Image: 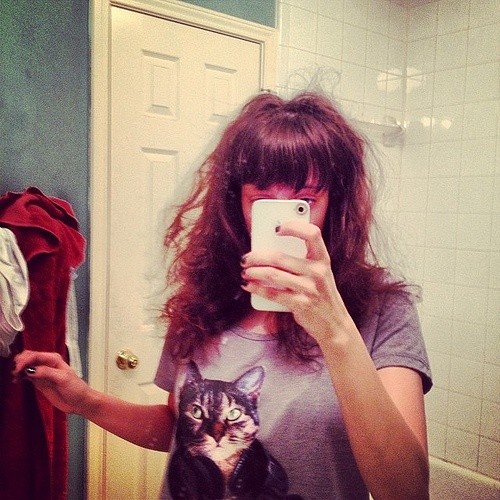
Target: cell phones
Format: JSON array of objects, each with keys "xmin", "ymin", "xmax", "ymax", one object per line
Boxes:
[{"xmin": 251, "ymin": 199, "xmax": 311, "ymax": 312}]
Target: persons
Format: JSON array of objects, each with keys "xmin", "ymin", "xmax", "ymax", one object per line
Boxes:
[{"xmin": 11, "ymin": 92, "xmax": 433, "ymax": 500}]
